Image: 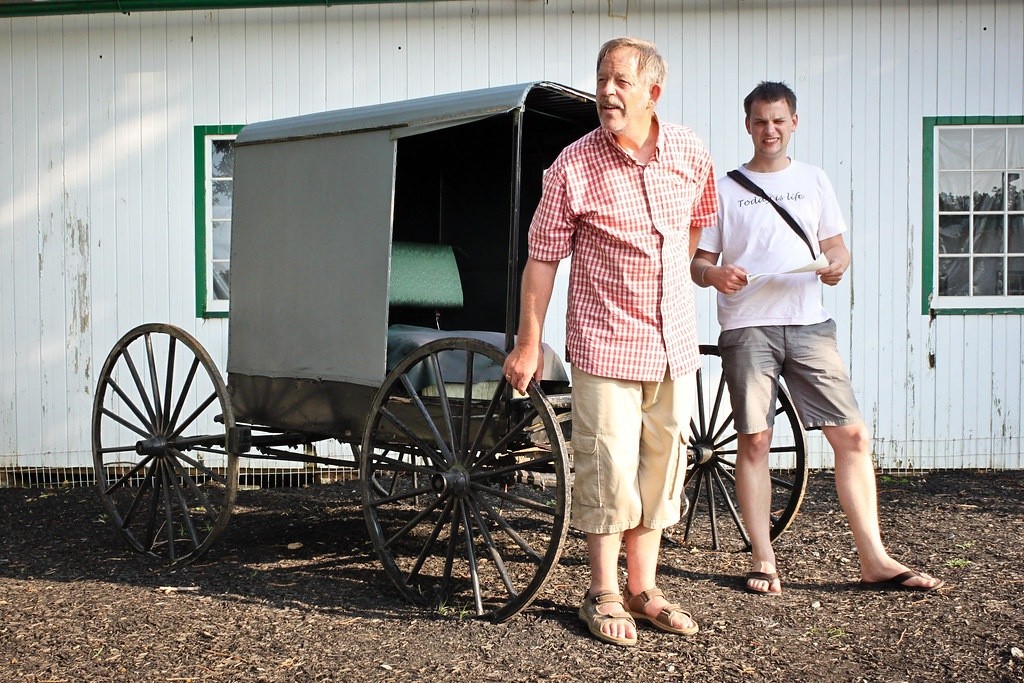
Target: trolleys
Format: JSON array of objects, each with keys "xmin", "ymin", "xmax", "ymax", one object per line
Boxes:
[{"xmin": 89, "ymin": 79, "xmax": 808, "ymax": 629}]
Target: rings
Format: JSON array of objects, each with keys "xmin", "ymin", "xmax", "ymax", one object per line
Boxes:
[{"xmin": 506, "ymin": 373, "xmax": 511, "ymax": 378}]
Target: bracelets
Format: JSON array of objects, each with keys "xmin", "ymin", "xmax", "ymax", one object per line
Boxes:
[{"xmin": 702, "ymin": 266, "xmax": 715, "ymax": 287}]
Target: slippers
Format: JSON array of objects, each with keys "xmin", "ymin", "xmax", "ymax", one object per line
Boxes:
[
  {"xmin": 859, "ymin": 566, "xmax": 944, "ymax": 591},
  {"xmin": 745, "ymin": 571, "xmax": 782, "ymax": 596}
]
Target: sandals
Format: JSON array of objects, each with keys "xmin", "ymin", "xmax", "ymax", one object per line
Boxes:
[
  {"xmin": 622, "ymin": 580, "xmax": 700, "ymax": 635},
  {"xmin": 578, "ymin": 588, "xmax": 638, "ymax": 646}
]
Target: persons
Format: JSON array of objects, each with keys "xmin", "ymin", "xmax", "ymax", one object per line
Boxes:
[
  {"xmin": 502, "ymin": 39, "xmax": 717, "ymax": 645},
  {"xmin": 691, "ymin": 81, "xmax": 944, "ymax": 596}
]
